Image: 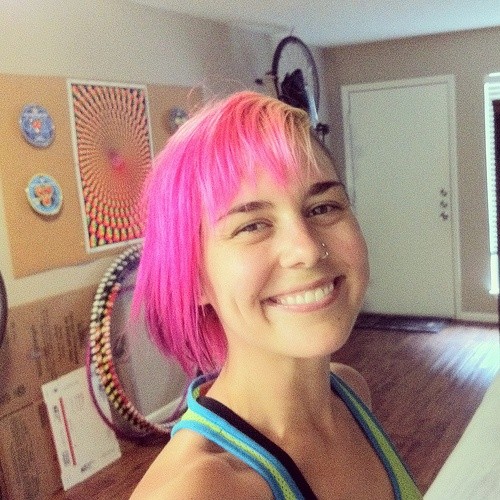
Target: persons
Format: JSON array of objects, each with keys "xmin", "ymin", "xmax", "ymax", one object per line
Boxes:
[{"xmin": 126, "ymin": 92, "xmax": 425, "ymax": 500}]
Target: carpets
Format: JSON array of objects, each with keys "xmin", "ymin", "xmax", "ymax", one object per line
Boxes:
[{"xmin": 355, "ymin": 311, "xmax": 451, "ymax": 334}]
